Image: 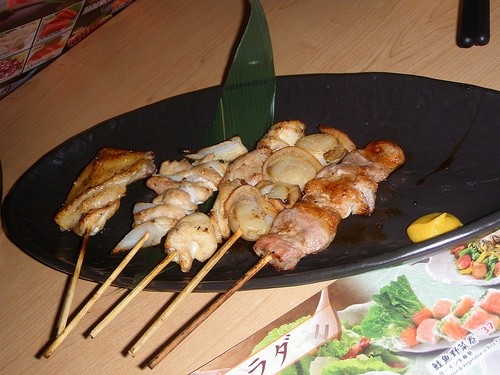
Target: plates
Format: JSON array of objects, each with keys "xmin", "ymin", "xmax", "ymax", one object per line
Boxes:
[
  {"xmin": 337, "ymin": 285, "xmax": 500, "ymax": 353},
  {"xmin": 0, "ymin": 73, "xmax": 500, "ymax": 293},
  {"xmin": 426, "ymin": 233, "xmax": 500, "ymax": 286}
]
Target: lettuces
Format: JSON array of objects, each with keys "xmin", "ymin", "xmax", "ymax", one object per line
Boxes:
[{"xmin": 249, "ymin": 276, "xmax": 429, "ymax": 375}]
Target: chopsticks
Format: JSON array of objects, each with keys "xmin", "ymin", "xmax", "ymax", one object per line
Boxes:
[{"xmin": 458, "ymin": 0, "xmax": 490, "ymax": 48}]
